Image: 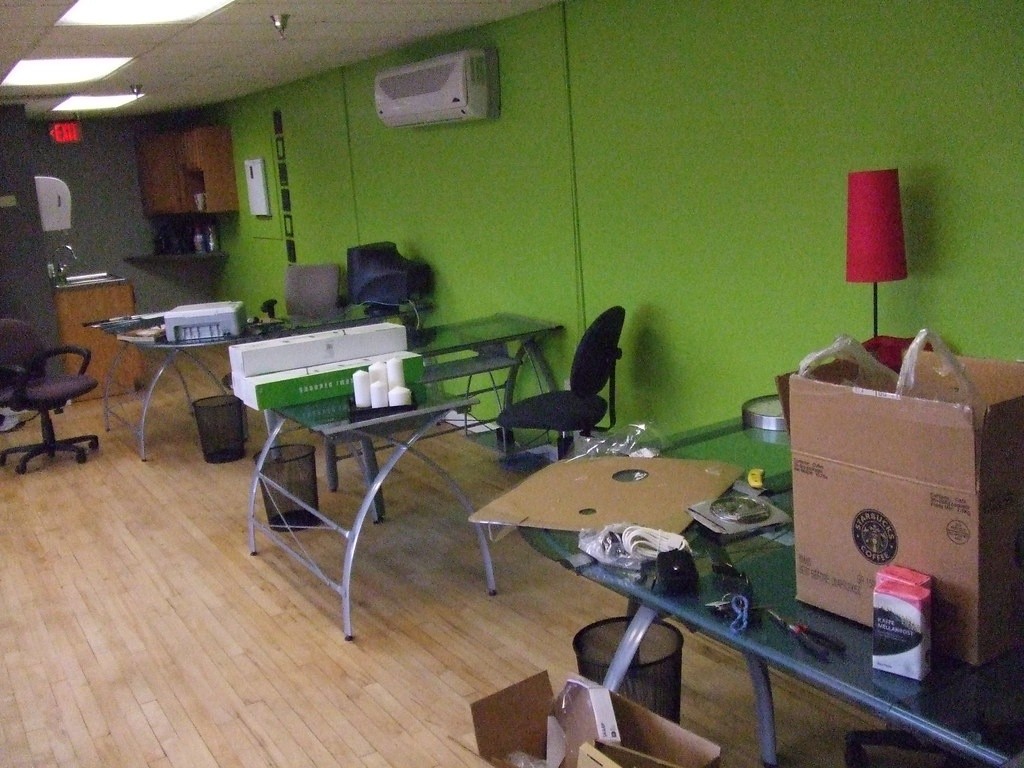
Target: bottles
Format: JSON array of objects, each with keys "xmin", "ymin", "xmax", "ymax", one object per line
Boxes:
[
  {"xmin": 194, "ymin": 226, "xmax": 206, "ymax": 254},
  {"xmin": 207, "ymin": 217, "xmax": 220, "ymax": 252}
]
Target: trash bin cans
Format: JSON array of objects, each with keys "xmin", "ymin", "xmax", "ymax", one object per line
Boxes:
[
  {"xmin": 251, "ymin": 444, "xmax": 321, "ymax": 531},
  {"xmin": 193, "ymin": 395, "xmax": 244, "ymax": 463},
  {"xmin": 574, "ymin": 616, "xmax": 683, "ymax": 722}
]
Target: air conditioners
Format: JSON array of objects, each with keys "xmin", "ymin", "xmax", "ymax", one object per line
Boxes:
[{"xmin": 374, "ymin": 46, "xmax": 500, "ymax": 128}]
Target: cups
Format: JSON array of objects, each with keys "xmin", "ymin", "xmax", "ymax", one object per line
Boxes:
[{"xmin": 194, "ymin": 194, "xmax": 205, "ymax": 210}]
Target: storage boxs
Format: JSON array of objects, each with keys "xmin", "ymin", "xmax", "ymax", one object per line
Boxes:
[
  {"xmin": 230, "ymin": 322, "xmax": 425, "ymax": 410},
  {"xmin": 773, "ymin": 350, "xmax": 1024, "ymax": 665},
  {"xmin": 447, "ymin": 669, "xmax": 722, "ymax": 768}
]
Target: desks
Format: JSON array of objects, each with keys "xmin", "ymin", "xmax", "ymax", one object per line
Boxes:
[
  {"xmin": 103, "ymin": 334, "xmax": 239, "ymax": 462},
  {"xmin": 304, "ymin": 313, "xmax": 569, "ymax": 523},
  {"xmin": 247, "ymin": 383, "xmax": 498, "ymax": 642},
  {"xmin": 516, "ymin": 415, "xmax": 1023, "ymax": 768}
]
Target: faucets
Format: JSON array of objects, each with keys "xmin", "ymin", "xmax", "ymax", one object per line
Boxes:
[{"xmin": 54, "ymin": 245, "xmax": 78, "ymax": 281}]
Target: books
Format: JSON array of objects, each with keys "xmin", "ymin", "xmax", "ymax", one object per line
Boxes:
[{"xmin": 116, "ymin": 324, "xmax": 166, "ymax": 342}]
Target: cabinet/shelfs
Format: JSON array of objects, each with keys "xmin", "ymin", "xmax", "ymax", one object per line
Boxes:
[
  {"xmin": 139, "ymin": 123, "xmax": 238, "ymax": 215},
  {"xmin": 54, "ymin": 272, "xmax": 146, "ymax": 403}
]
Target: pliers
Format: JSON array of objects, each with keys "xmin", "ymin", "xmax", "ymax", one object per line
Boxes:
[{"xmin": 767, "ymin": 607, "xmax": 847, "ymax": 658}]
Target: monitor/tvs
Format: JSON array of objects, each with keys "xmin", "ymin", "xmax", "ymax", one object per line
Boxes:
[{"xmin": 347, "ymin": 241, "xmax": 433, "ymax": 317}]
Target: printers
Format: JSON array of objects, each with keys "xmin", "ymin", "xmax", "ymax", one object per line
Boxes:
[{"xmin": 163, "ymin": 301, "xmax": 247, "ymax": 344}]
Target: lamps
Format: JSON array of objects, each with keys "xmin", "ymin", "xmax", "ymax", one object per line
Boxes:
[{"xmin": 847, "ymin": 168, "xmax": 909, "ymax": 337}]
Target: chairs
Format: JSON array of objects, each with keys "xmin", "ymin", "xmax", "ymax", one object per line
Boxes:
[
  {"xmin": 496, "ymin": 305, "xmax": 626, "ymax": 459},
  {"xmin": 0, "ymin": 318, "xmax": 98, "ymax": 474},
  {"xmin": 261, "ymin": 264, "xmax": 342, "ymax": 330}
]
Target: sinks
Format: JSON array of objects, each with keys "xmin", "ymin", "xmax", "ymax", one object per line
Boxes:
[{"xmin": 65, "ymin": 272, "xmax": 125, "ymax": 286}]
